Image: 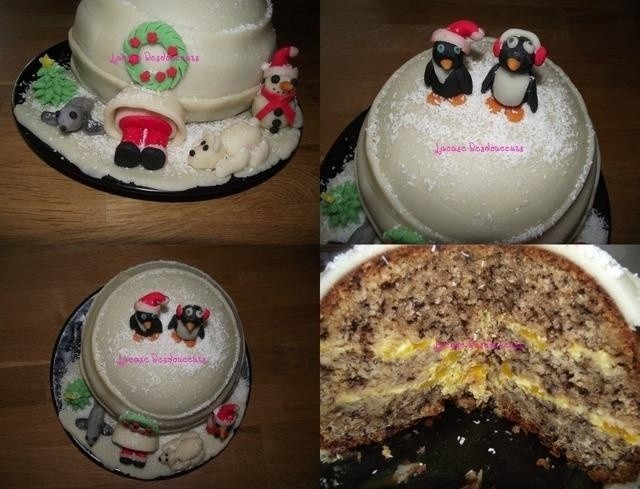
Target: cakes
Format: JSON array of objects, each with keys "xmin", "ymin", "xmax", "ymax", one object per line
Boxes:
[
  {"xmin": 12, "ymin": 0, "xmax": 304, "ymax": 193},
  {"xmin": 320, "ymin": 245, "xmax": 640, "ymax": 489},
  {"xmin": 321, "ymin": 18, "xmax": 603, "ymax": 245},
  {"xmin": 57, "ymin": 260, "xmax": 251, "ymax": 480}
]
